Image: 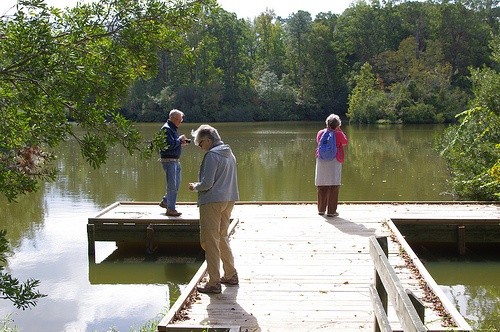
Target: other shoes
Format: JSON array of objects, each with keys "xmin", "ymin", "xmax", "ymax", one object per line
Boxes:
[
  {"xmin": 319, "ymin": 212, "xmax": 339, "ymax": 216},
  {"xmin": 158, "ymin": 201, "xmax": 167, "ymax": 209},
  {"xmin": 166, "ymin": 209, "xmax": 182, "ymax": 215}
]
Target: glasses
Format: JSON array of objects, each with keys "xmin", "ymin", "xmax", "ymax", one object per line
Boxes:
[{"xmin": 171, "ymin": 117, "xmax": 184, "ymax": 122}]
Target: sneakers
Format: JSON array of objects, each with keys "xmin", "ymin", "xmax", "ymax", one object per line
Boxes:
[
  {"xmin": 220, "ymin": 277, "xmax": 238, "ymax": 285},
  {"xmin": 196, "ymin": 283, "xmax": 221, "ymax": 293}
]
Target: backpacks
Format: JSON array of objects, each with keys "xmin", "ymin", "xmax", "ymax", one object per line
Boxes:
[{"xmin": 319, "ymin": 128, "xmax": 337, "ymax": 161}]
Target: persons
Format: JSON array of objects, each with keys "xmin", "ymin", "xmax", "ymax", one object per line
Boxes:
[
  {"xmin": 314, "ymin": 113, "xmax": 350, "ymax": 217},
  {"xmin": 158, "ymin": 109, "xmax": 189, "ymax": 217},
  {"xmin": 188, "ymin": 123, "xmax": 239, "ymax": 294}
]
